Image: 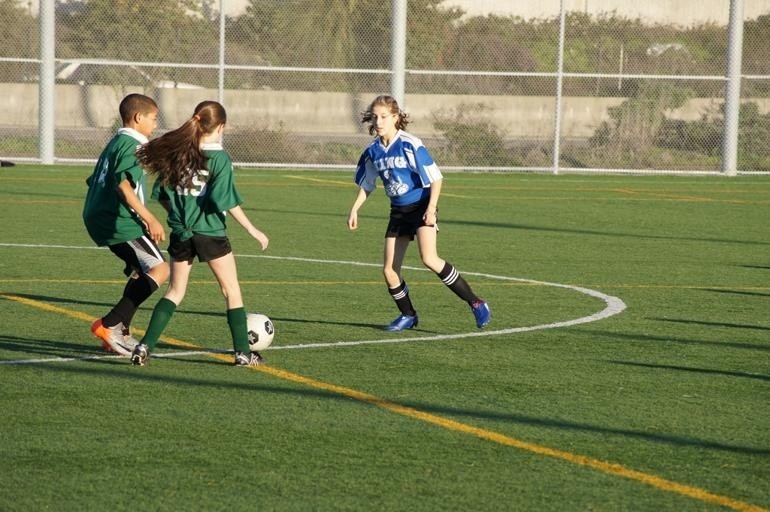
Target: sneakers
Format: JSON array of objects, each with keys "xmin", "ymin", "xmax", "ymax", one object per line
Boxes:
[
  {"xmin": 381, "ymin": 313, "xmax": 419, "ymax": 332},
  {"xmin": 130, "ymin": 344, "xmax": 151, "ymax": 368},
  {"xmin": 234, "ymin": 350, "xmax": 270, "ymax": 367},
  {"xmin": 90, "ymin": 317, "xmax": 141, "ymax": 355},
  {"xmin": 472, "ymin": 300, "xmax": 492, "ymax": 329}
]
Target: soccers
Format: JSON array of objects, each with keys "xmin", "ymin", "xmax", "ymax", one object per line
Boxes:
[{"xmin": 246, "ymin": 313, "xmax": 274, "ymax": 351}]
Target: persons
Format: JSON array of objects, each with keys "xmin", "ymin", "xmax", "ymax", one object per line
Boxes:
[
  {"xmin": 81, "ymin": 92, "xmax": 174, "ymax": 360},
  {"xmin": 130, "ymin": 99, "xmax": 272, "ymax": 366},
  {"xmin": 347, "ymin": 94, "xmax": 493, "ymax": 330}
]
